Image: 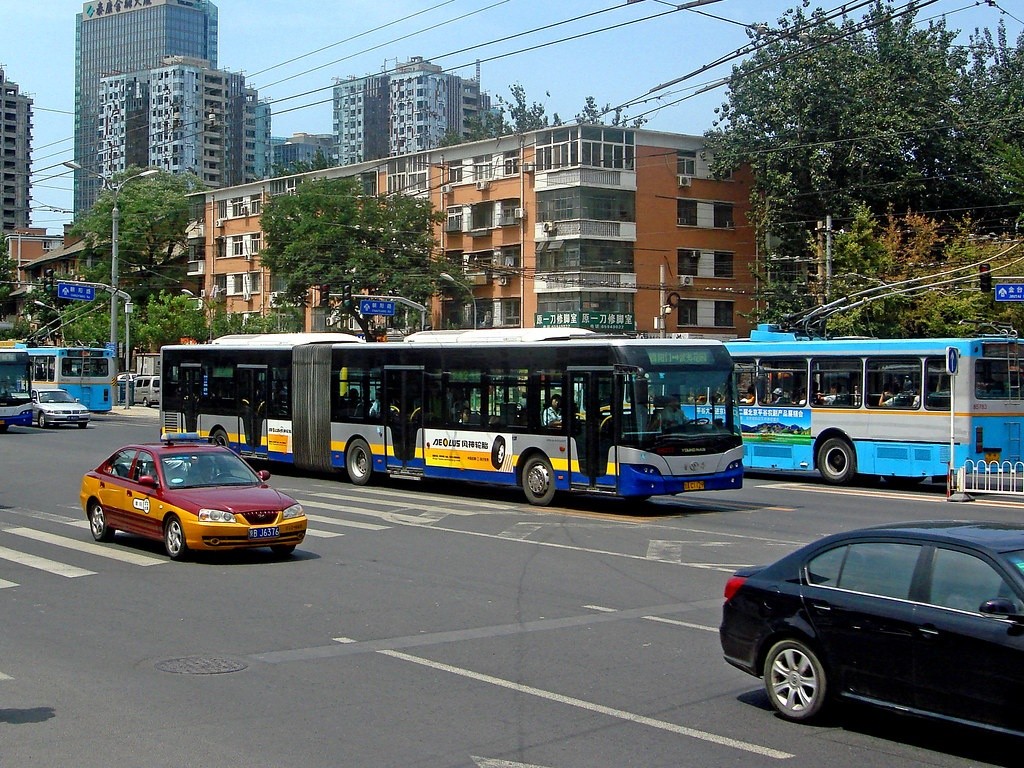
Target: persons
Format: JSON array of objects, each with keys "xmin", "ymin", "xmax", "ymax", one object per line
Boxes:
[
  {"xmin": 347, "ymin": 388, "xmax": 359, "ymax": 406},
  {"xmin": 543, "ymin": 394, "xmax": 562, "ymax": 428},
  {"xmin": 770, "ymin": 387, "xmax": 806, "ymax": 404},
  {"xmin": 818, "ymin": 381, "xmax": 842, "ymax": 405},
  {"xmin": 520, "ymin": 392, "xmax": 526, "ymax": 407},
  {"xmin": 369, "ymin": 388, "xmax": 381, "ymax": 418},
  {"xmin": 459, "ymin": 407, "xmax": 471, "ymax": 424},
  {"xmin": 656, "ymin": 394, "xmax": 685, "ymax": 429},
  {"xmin": 878, "ymin": 385, "xmax": 920, "ymax": 407},
  {"xmin": 903, "ymin": 376, "xmax": 912, "ymax": 390},
  {"xmin": 196, "ymin": 459, "xmax": 213, "ymax": 483},
  {"xmin": 745, "ymin": 384, "xmax": 755, "ymax": 404}
]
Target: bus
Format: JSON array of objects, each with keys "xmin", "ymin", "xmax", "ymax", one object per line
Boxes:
[
  {"xmin": 0, "ymin": 339, "xmax": 115, "ymax": 410},
  {"xmin": 0, "ymin": 348, "xmax": 35, "ymax": 433},
  {"xmin": 573, "ymin": 323, "xmax": 1024, "ymax": 485},
  {"xmin": 159, "ymin": 333, "xmax": 744, "ymax": 506}
]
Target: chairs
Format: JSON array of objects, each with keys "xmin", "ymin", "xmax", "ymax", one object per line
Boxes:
[{"xmin": 145, "ymin": 460, "xmax": 170, "ymax": 483}]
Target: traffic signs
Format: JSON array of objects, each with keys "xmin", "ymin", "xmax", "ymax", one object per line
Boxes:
[
  {"xmin": 58, "ymin": 283, "xmax": 95, "ymax": 301},
  {"xmin": 360, "ymin": 300, "xmax": 395, "ymax": 316},
  {"xmin": 995, "ymin": 283, "xmax": 1024, "ymax": 302}
]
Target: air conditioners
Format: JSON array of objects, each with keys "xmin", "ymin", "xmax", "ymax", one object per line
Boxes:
[
  {"xmin": 680, "ymin": 275, "xmax": 694, "ymax": 287},
  {"xmin": 441, "ymin": 184, "xmax": 452, "ymax": 193},
  {"xmin": 690, "ymin": 251, "xmax": 701, "ymax": 258},
  {"xmin": 724, "ymin": 221, "xmax": 735, "ymax": 229},
  {"xmin": 240, "ymin": 206, "xmax": 248, "ymax": 214},
  {"xmin": 201, "ymin": 290, "xmax": 205, "ymax": 297},
  {"xmin": 677, "ymin": 176, "xmax": 691, "ymax": 187},
  {"xmin": 498, "ymin": 276, "xmax": 509, "ymax": 285},
  {"xmin": 326, "ymin": 318, "xmax": 334, "ymax": 325},
  {"xmin": 542, "ymin": 222, "xmax": 556, "ymax": 232},
  {"xmin": 215, "ymin": 220, "xmax": 224, "ymax": 227},
  {"xmin": 677, "ymin": 218, "xmax": 686, "ymax": 224},
  {"xmin": 476, "ymin": 180, "xmax": 487, "ymax": 190},
  {"xmin": 245, "ymin": 254, "xmax": 252, "ymax": 259},
  {"xmin": 523, "ymin": 162, "xmax": 533, "ymax": 171},
  {"xmin": 243, "ymin": 293, "xmax": 252, "ymax": 300},
  {"xmin": 515, "ymin": 208, "xmax": 526, "ymax": 218}
]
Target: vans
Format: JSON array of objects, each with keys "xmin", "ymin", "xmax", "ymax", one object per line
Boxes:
[{"xmin": 134, "ymin": 376, "xmax": 161, "ymax": 408}]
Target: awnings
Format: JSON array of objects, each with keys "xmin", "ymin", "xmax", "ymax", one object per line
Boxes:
[
  {"xmin": 494, "ymin": 242, "xmax": 520, "ymax": 247},
  {"xmin": 461, "ymin": 248, "xmax": 493, "ymax": 255},
  {"xmin": 443, "ymin": 249, "xmax": 464, "ymax": 252},
  {"xmin": 470, "ymin": 198, "xmax": 519, "ymax": 206}
]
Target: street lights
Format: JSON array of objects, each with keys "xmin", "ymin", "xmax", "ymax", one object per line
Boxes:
[
  {"xmin": 440, "ymin": 273, "xmax": 476, "ymax": 331},
  {"xmin": 33, "ymin": 300, "xmax": 64, "ymax": 346},
  {"xmin": 182, "ymin": 289, "xmax": 212, "ymax": 339},
  {"xmin": 64, "ymin": 162, "xmax": 160, "ymax": 409}
]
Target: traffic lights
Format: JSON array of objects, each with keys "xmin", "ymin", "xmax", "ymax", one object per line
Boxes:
[
  {"xmin": 340, "ymin": 283, "xmax": 352, "ymax": 315},
  {"xmin": 979, "ymin": 263, "xmax": 992, "ymax": 293},
  {"xmin": 43, "ymin": 268, "xmax": 54, "ymax": 295},
  {"xmin": 320, "ymin": 284, "xmax": 330, "ymax": 309}
]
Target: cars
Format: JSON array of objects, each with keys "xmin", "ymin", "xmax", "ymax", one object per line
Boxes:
[
  {"xmin": 80, "ymin": 442, "xmax": 308, "ymax": 559},
  {"xmin": 720, "ymin": 519, "xmax": 1024, "ymax": 741},
  {"xmin": 31, "ymin": 388, "xmax": 91, "ymax": 429}
]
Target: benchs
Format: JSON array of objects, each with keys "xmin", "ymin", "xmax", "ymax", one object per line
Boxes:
[{"xmin": 117, "ymin": 460, "xmax": 186, "ymax": 484}]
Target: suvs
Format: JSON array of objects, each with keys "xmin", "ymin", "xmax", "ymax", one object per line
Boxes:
[{"xmin": 117, "ymin": 372, "xmax": 137, "ymax": 381}]
